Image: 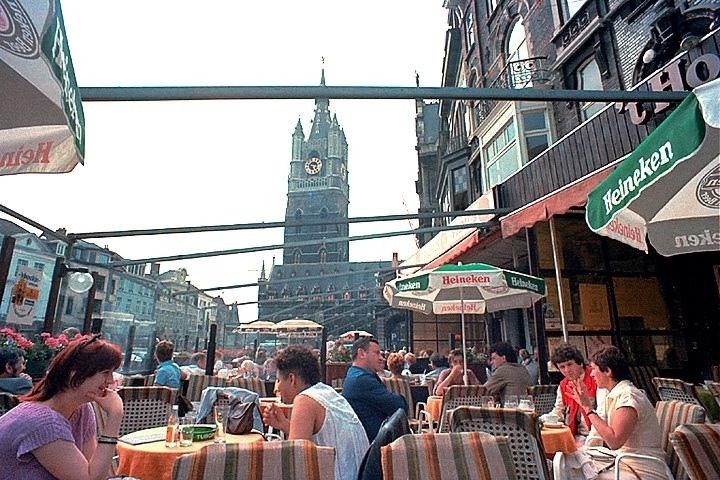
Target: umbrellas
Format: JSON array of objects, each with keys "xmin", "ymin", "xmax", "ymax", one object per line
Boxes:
[
  {"xmin": 233, "ymin": 319, "xmax": 278, "ymax": 343},
  {"xmin": 0, "ymin": 0, "xmax": 86, "ymax": 175},
  {"xmin": 340, "ymin": 331, "xmax": 374, "ymax": 341},
  {"xmin": 271, "ymin": 317, "xmax": 323, "ymax": 341},
  {"xmin": 383, "ymin": 262, "xmax": 548, "ymax": 384},
  {"xmin": 586, "ymin": 81, "xmax": 718, "ymax": 255}
]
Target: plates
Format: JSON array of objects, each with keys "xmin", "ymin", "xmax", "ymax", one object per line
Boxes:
[
  {"xmin": 703, "ymin": 385, "xmax": 710, "ymax": 388},
  {"xmin": 545, "ymin": 422, "xmax": 565, "ymax": 428}
]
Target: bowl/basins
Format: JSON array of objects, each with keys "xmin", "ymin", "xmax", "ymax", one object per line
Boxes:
[{"xmin": 184, "ymin": 426, "xmax": 216, "ymax": 440}]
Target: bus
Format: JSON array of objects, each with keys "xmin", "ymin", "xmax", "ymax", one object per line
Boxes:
[{"xmin": 259, "ymin": 339, "xmax": 288, "ymax": 351}]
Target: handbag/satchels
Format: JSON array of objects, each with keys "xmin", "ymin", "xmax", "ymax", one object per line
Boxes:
[
  {"xmin": 226, "ymin": 398, "xmax": 255, "ymax": 435},
  {"xmin": 171, "ymin": 382, "xmax": 192, "ymax": 418}
]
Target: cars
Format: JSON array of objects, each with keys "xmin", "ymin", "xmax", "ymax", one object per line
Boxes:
[{"xmin": 119, "ymin": 348, "xmax": 181, "ymax": 369}]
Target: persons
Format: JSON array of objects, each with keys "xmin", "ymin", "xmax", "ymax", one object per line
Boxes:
[
  {"xmin": 0, "ymin": 346, "xmax": 33, "ymax": 398},
  {"xmin": 1, "ymin": 334, "xmax": 126, "ymax": 480}
]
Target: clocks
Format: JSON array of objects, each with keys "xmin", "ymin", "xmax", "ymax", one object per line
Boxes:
[{"xmin": 304, "ymin": 157, "xmax": 323, "ymax": 175}]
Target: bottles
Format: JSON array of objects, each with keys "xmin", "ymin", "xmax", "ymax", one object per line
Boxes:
[{"xmin": 166, "ymin": 405, "xmax": 178, "ymax": 448}]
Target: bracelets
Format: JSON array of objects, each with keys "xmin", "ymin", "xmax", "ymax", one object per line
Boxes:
[{"xmin": 96, "ymin": 435, "xmax": 118, "ymax": 444}]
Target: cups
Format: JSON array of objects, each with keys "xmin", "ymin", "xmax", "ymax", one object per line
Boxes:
[
  {"xmin": 546, "ymin": 414, "xmax": 560, "ymax": 425},
  {"xmin": 488, "ymin": 395, "xmax": 533, "ymax": 413},
  {"xmin": 704, "ymin": 381, "xmax": 713, "ymax": 386},
  {"xmin": 180, "ymin": 417, "xmax": 196, "ymax": 446},
  {"xmin": 404, "ymin": 372, "xmax": 426, "ymax": 381},
  {"xmin": 214, "ymin": 405, "xmax": 229, "ymax": 442}
]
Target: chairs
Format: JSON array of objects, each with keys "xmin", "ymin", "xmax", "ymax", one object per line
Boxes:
[
  {"xmin": 628, "ymin": 366, "xmax": 720, "ymax": 480},
  {"xmin": 358, "ymin": 377, "xmax": 572, "ymax": 480},
  {"xmin": 171, "ymin": 439, "xmax": 319, "ymax": 480},
  {"xmin": 117, "ymin": 373, "xmax": 268, "ymax": 434}
]
